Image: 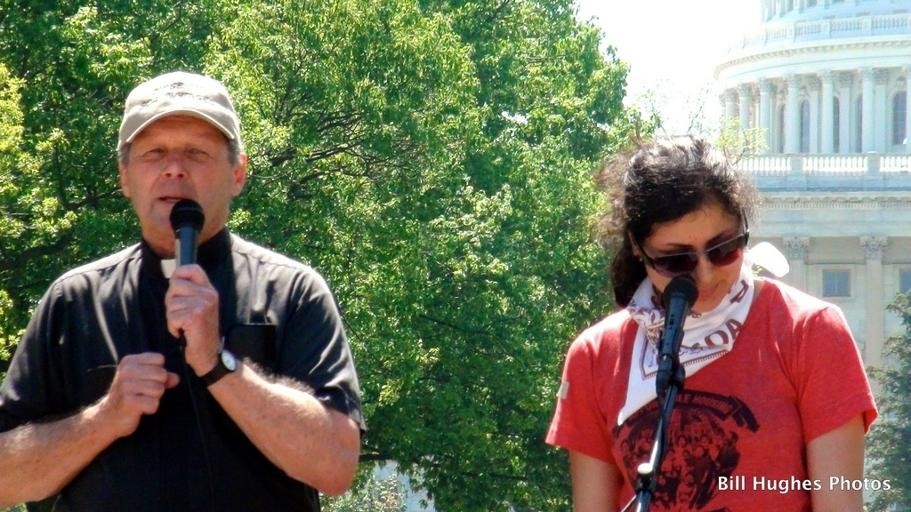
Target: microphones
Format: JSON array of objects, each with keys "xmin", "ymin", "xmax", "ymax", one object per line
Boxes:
[
  {"xmin": 169, "ymin": 197, "xmax": 205, "ymax": 267},
  {"xmin": 655, "ymin": 277, "xmax": 698, "ymax": 387}
]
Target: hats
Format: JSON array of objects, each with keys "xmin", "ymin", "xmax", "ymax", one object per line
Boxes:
[{"xmin": 119, "ymin": 71, "xmax": 241, "ymax": 146}]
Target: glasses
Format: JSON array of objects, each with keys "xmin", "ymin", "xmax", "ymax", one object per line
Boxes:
[{"xmin": 628, "ymin": 206, "xmax": 750, "ymax": 280}]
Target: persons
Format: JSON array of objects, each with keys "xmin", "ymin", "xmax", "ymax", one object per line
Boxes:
[
  {"xmin": 0, "ymin": 68, "xmax": 368, "ymax": 512},
  {"xmin": 543, "ymin": 135, "xmax": 876, "ymax": 512}
]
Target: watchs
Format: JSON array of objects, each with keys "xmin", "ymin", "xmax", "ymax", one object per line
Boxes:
[{"xmin": 198, "ymin": 348, "xmax": 237, "ymax": 387}]
